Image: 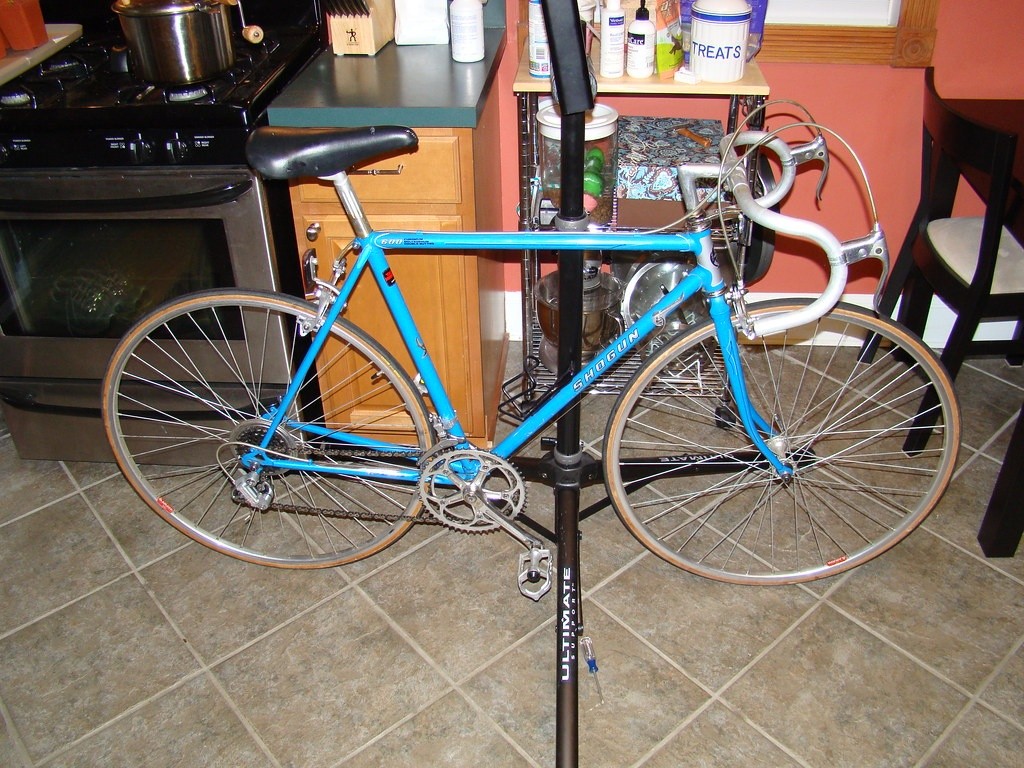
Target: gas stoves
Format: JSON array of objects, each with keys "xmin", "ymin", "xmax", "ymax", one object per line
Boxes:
[{"xmin": 0, "ymin": 20, "xmax": 318, "ymax": 167}]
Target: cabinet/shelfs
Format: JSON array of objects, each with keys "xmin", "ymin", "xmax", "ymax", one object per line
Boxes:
[
  {"xmin": 266, "ymin": 72, "xmax": 510, "ymax": 457},
  {"xmin": 512, "ymin": 36, "xmax": 771, "ymax": 433}
]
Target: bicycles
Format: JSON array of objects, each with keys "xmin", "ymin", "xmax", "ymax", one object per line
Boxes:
[{"xmin": 101, "ymin": 98, "xmax": 964, "ymax": 600}]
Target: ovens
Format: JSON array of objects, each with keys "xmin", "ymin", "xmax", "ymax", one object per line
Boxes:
[{"xmin": 1, "ymin": 170, "xmax": 295, "ymax": 384}]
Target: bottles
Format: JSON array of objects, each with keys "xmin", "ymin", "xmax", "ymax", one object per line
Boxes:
[
  {"xmin": 599, "ymin": 0, "xmax": 625, "ymax": 79},
  {"xmin": 450, "ymin": 0, "xmax": 485, "ymax": 62},
  {"xmin": 528, "ymin": 1, "xmax": 552, "ymax": 78},
  {"xmin": 627, "ymin": 0, "xmax": 656, "ymax": 78}
]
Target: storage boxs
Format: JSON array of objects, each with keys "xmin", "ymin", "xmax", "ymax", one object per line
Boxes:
[{"xmin": 619, "ymin": 115, "xmax": 735, "ymax": 227}]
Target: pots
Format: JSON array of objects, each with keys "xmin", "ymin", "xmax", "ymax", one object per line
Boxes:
[
  {"xmin": 110, "ymin": 0, "xmax": 238, "ymax": 87},
  {"xmin": 534, "ymin": 248, "xmax": 626, "ymax": 377}
]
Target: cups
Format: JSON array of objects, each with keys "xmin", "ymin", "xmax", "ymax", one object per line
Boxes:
[{"xmin": 689, "ymin": 0, "xmax": 753, "ymax": 82}]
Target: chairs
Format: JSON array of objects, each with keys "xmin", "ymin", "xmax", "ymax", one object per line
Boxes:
[{"xmin": 857, "ymin": 66, "xmax": 1024, "ymax": 457}]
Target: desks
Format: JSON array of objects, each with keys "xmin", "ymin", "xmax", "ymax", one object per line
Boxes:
[{"xmin": 893, "ymin": 96, "xmax": 1024, "ymax": 560}]
